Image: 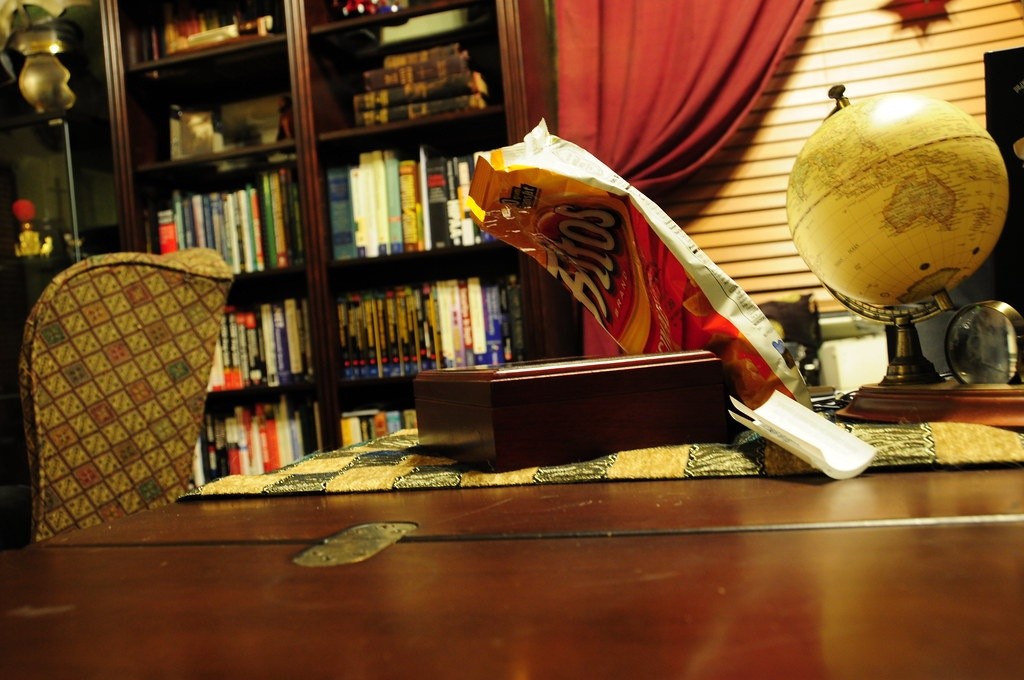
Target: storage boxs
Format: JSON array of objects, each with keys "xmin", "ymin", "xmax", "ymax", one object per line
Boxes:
[{"xmin": 413, "ymin": 349, "xmax": 727, "ymax": 476}]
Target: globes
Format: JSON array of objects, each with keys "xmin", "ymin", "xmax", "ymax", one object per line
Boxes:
[{"xmin": 781, "ymin": 83, "xmax": 1011, "ymax": 395}]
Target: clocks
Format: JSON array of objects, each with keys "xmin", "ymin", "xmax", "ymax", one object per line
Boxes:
[{"xmin": 943, "ymin": 301, "xmax": 1024, "ymax": 385}]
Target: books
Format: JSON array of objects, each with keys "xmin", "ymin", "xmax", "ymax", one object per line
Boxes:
[
  {"xmin": 151, "ymin": 148, "xmax": 535, "ymax": 490},
  {"xmin": 164, "ymin": 12, "xmax": 278, "ymax": 56},
  {"xmin": 353, "ymin": 42, "xmax": 488, "ymax": 127}
]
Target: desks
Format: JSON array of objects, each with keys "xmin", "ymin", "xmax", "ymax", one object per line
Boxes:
[{"xmin": 2, "ymin": 467, "xmax": 1024, "ymax": 680}]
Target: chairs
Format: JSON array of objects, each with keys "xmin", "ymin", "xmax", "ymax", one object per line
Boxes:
[{"xmin": 17, "ymin": 246, "xmax": 236, "ymax": 548}]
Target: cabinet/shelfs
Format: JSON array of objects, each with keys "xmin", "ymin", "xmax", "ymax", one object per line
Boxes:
[{"xmin": 101, "ymin": 0, "xmax": 584, "ymax": 461}]
